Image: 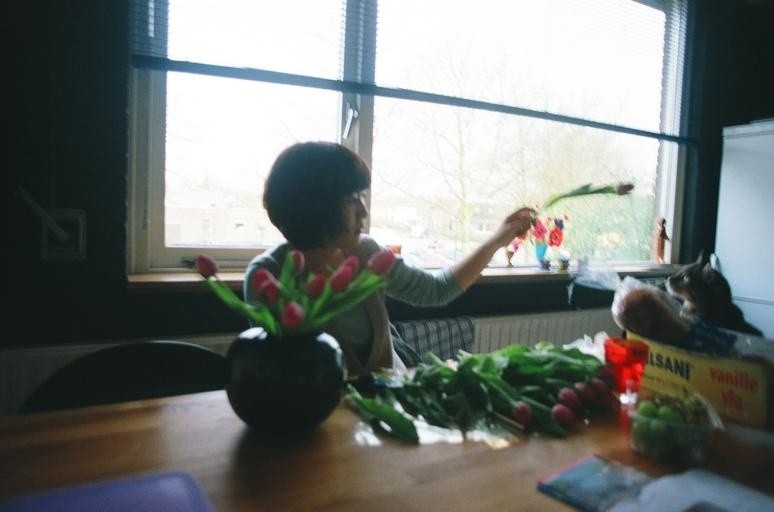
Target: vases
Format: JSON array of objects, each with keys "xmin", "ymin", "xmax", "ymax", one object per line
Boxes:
[{"xmin": 223, "ymin": 327, "xmax": 353, "ymax": 437}]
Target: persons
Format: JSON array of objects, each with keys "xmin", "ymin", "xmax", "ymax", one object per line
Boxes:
[
  {"xmin": 237, "ymin": 138, "xmax": 534, "ymax": 383},
  {"xmin": 654, "ymin": 216, "xmax": 669, "ymax": 263}
]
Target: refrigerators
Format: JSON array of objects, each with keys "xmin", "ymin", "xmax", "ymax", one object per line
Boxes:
[{"xmin": 710, "ymin": 118, "xmax": 773, "ymax": 343}]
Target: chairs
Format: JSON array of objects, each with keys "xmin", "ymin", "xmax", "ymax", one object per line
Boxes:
[{"xmin": 14, "ymin": 341, "xmax": 227, "ymax": 425}]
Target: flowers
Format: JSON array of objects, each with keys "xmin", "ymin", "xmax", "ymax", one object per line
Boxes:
[{"xmin": 181, "ymin": 246, "xmax": 402, "ymax": 336}]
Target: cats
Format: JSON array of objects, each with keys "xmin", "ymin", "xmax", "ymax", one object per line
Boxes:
[{"xmin": 665, "ymin": 248, "xmax": 765, "ymax": 339}]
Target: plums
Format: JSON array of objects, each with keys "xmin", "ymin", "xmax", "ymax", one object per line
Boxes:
[{"xmin": 631, "ymin": 393, "xmax": 704, "ymax": 443}]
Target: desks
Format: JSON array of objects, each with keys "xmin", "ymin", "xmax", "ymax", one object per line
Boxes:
[{"xmin": 1, "ymin": 342, "xmax": 774, "ymax": 512}]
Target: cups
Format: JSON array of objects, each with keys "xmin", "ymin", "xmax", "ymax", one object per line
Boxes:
[
  {"xmin": 558, "ymin": 258, "xmax": 571, "ymax": 269},
  {"xmin": 603, "ymin": 338, "xmax": 650, "ymax": 405}
]
